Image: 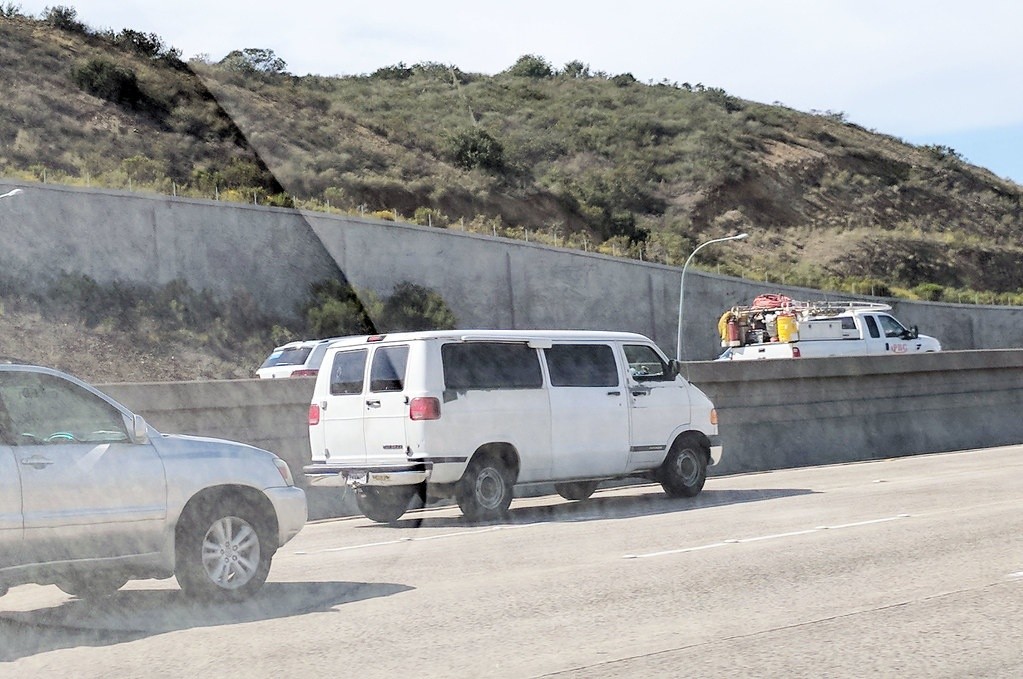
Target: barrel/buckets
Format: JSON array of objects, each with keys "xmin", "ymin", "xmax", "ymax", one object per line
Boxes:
[{"xmin": 777, "ymin": 314, "xmax": 797, "ymax": 342}]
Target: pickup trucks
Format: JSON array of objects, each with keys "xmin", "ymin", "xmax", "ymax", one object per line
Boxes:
[{"xmin": 715, "ymin": 293, "xmax": 943, "ymax": 360}]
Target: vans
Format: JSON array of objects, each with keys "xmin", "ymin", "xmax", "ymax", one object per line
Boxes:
[{"xmin": 305, "ymin": 326, "xmax": 724, "ymax": 522}]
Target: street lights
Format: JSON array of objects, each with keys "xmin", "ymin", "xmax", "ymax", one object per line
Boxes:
[{"xmin": 675, "ymin": 233, "xmax": 747, "ymax": 361}]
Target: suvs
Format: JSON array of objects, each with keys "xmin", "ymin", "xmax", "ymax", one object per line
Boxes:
[
  {"xmin": 255, "ymin": 337, "xmax": 339, "ymax": 382},
  {"xmin": 1, "ymin": 364, "xmax": 308, "ymax": 606}
]
